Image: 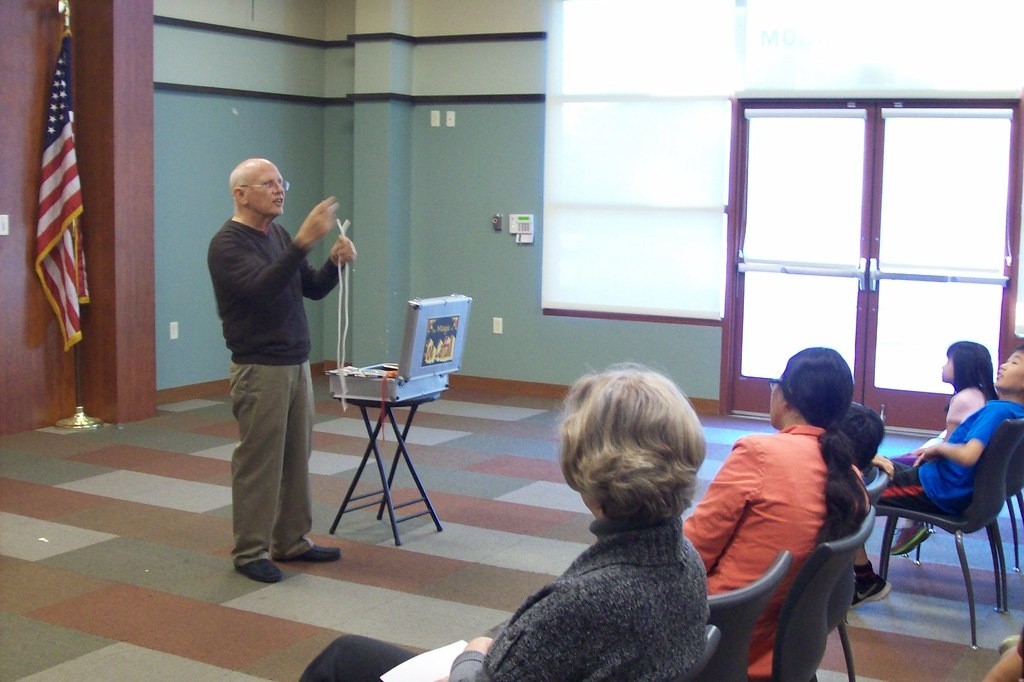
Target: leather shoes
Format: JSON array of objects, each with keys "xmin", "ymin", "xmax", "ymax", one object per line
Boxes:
[
  {"xmin": 294, "ymin": 545, "xmax": 339, "ymax": 562},
  {"xmin": 236, "ymin": 559, "xmax": 282, "ymax": 582}
]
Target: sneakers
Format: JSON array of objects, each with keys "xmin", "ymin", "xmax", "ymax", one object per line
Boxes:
[
  {"xmin": 848, "ymin": 574, "xmax": 892, "ymax": 608},
  {"xmin": 890, "ymin": 523, "xmax": 930, "ymax": 555}
]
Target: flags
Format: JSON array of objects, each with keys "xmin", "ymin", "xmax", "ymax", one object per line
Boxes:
[{"xmin": 34, "ymin": 32, "xmax": 89, "ymax": 353}]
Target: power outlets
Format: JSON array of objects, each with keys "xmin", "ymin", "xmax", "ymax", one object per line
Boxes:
[
  {"xmin": 493, "ymin": 318, "xmax": 503, "ymax": 334},
  {"xmin": 170, "ymin": 322, "xmax": 179, "ymax": 340}
]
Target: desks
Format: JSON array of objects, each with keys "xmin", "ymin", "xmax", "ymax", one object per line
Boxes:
[{"xmin": 328, "ymin": 390, "xmax": 441, "ymax": 546}]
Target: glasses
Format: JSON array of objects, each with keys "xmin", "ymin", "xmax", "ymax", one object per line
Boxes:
[
  {"xmin": 240, "ymin": 181, "xmax": 289, "ymax": 191},
  {"xmin": 769, "ymin": 378, "xmax": 784, "ymax": 389}
]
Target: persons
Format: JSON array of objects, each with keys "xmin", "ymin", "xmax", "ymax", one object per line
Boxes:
[
  {"xmin": 851, "ymin": 345, "xmax": 1024, "ymax": 608},
  {"xmin": 208, "ymin": 158, "xmax": 358, "ymax": 583},
  {"xmin": 840, "ymin": 402, "xmax": 886, "ymax": 469},
  {"xmin": 299, "ymin": 358, "xmax": 711, "ymax": 682},
  {"xmin": 891, "ymin": 341, "xmax": 998, "ymax": 555},
  {"xmin": 682, "ymin": 348, "xmax": 869, "ymax": 681},
  {"xmin": 982, "ymin": 626, "xmax": 1024, "ymax": 682}
]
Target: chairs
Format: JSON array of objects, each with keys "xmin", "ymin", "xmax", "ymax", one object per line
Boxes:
[{"xmin": 676, "ymin": 415, "xmax": 1024, "ymax": 682}]
